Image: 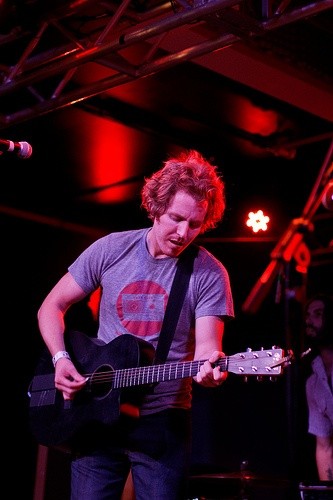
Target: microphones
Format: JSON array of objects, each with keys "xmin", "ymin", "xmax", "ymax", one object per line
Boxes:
[{"xmin": 0, "ymin": 138, "xmax": 32, "ymax": 159}]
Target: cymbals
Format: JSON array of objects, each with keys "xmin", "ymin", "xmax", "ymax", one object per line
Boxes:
[{"xmin": 188, "ymin": 469, "xmax": 267, "ymax": 481}]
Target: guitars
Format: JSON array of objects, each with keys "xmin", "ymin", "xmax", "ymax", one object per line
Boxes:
[{"xmin": 28, "ymin": 330, "xmax": 294, "ymax": 442}]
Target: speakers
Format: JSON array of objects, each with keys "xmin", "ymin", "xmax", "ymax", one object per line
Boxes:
[{"xmin": 289, "ymin": 480, "xmax": 333, "ymax": 500}]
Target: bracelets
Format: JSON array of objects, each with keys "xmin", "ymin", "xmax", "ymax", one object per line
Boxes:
[{"xmin": 52, "ymin": 350, "xmax": 71, "ymax": 367}]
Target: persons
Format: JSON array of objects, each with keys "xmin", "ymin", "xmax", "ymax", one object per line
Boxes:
[
  {"xmin": 304, "ymin": 294, "xmax": 333, "ymax": 480},
  {"xmin": 37, "ymin": 151, "xmax": 236, "ymax": 500}
]
